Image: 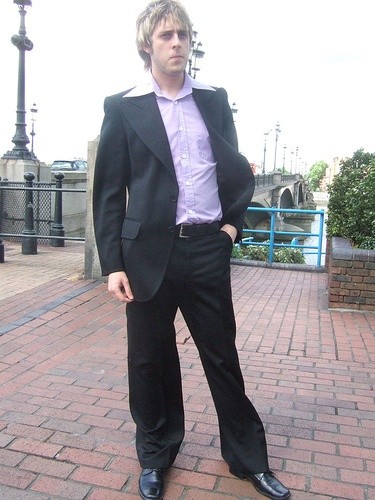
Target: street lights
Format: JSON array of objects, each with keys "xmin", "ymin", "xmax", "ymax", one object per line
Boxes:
[
  {"xmin": 282, "ymin": 142, "xmax": 308, "ymax": 179},
  {"xmin": 231, "ymin": 97, "xmax": 237, "ymax": 124},
  {"xmin": 274, "ymin": 115, "xmax": 282, "ymax": 174},
  {"xmin": 263, "ymin": 128, "xmax": 269, "ymax": 175},
  {"xmin": 2, "ymin": 0, "xmax": 42, "ymax": 160},
  {"xmin": 185, "ymin": 21, "xmax": 204, "ymax": 79}
]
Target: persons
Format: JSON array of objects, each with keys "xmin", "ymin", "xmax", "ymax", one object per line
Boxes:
[{"xmin": 93, "ymin": 0, "xmax": 291, "ymax": 500}]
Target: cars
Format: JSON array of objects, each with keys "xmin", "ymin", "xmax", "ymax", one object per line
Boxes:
[{"xmin": 50, "ymin": 160, "xmax": 88, "ymax": 173}]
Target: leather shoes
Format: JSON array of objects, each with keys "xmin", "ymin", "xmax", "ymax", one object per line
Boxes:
[
  {"xmin": 229, "ymin": 466, "xmax": 292, "ymax": 500},
  {"xmin": 139, "ymin": 469, "xmax": 164, "ymax": 500}
]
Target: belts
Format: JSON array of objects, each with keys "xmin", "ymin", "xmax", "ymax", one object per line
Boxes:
[{"xmin": 177, "ymin": 222, "xmax": 221, "ymax": 238}]
